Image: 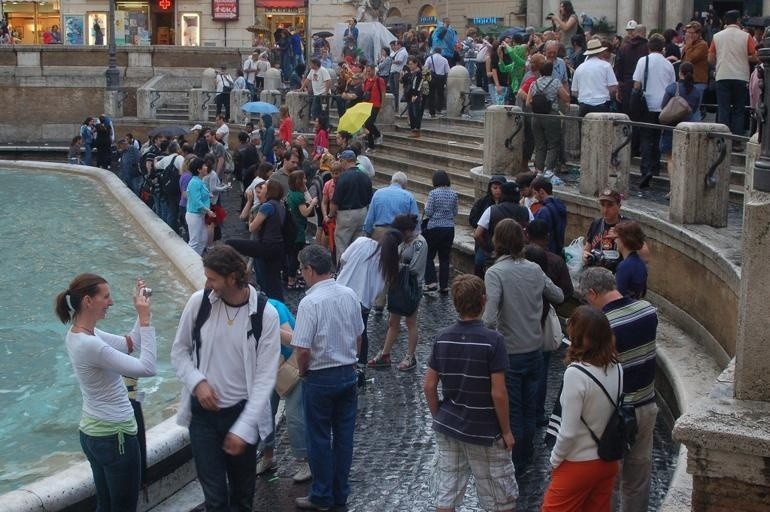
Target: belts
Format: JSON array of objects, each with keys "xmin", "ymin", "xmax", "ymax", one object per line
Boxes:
[{"xmin": 337, "ymin": 206, "xmax": 360, "ymax": 210}]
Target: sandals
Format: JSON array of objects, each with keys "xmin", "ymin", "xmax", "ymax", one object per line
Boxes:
[{"xmin": 287, "ymin": 279, "xmax": 306, "ymax": 290}]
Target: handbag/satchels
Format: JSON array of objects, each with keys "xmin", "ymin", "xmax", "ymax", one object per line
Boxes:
[
  {"xmin": 387, "ymin": 266, "xmax": 420, "ymax": 317},
  {"xmin": 658, "ymin": 96, "xmax": 692, "ymax": 125},
  {"xmin": 629, "ymin": 89, "xmax": 651, "ymax": 123},
  {"xmin": 362, "ymin": 91, "xmax": 370, "ymax": 101},
  {"xmin": 429, "ymin": 70, "xmax": 441, "ymax": 88}
]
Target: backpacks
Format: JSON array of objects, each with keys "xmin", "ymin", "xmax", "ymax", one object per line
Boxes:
[
  {"xmin": 597, "ymin": 392, "xmax": 639, "ymax": 463},
  {"xmin": 307, "ymin": 174, "xmax": 324, "ymax": 209},
  {"xmin": 137, "ymin": 155, "xmax": 148, "ymax": 176},
  {"xmin": 531, "ymin": 89, "xmax": 551, "ymax": 114},
  {"xmin": 161, "ymin": 164, "xmax": 181, "ymax": 197},
  {"xmin": 224, "ymin": 152, "xmax": 235, "ymax": 174}
]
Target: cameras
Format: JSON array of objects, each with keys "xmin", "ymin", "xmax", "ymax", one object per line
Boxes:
[
  {"xmin": 138, "ymin": 285, "xmax": 155, "ymax": 299},
  {"xmin": 586, "ymin": 250, "xmax": 604, "ymax": 267}
]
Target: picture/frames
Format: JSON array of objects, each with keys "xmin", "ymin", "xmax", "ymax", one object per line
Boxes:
[
  {"xmin": 212, "ymin": 0, "xmax": 239, "ymax": 21},
  {"xmin": 86, "ymin": 10, "xmax": 110, "ymax": 45},
  {"xmin": 179, "ymin": 10, "xmax": 201, "ymax": 46},
  {"xmin": 61, "ymin": 13, "xmax": 86, "ymax": 45}
]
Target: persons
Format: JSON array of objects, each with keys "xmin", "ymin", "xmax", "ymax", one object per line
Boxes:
[
  {"xmin": 579, "ymin": 267, "xmax": 659, "ymax": 511},
  {"xmin": 368, "ymin": 214, "xmax": 428, "ymax": 372},
  {"xmin": 56, "ymin": 273, "xmax": 158, "ymax": 512},
  {"xmin": 424, "ymin": 275, "xmax": 519, "ymax": 512},
  {"xmin": 337, "ymin": 229, "xmax": 404, "ymax": 375},
  {"xmin": 247, "ymin": 281, "xmax": 314, "ymax": 483},
  {"xmin": 51, "ymin": 26, "xmax": 63, "ymax": 44},
  {"xmin": 170, "ymin": 241, "xmax": 281, "ymax": 512},
  {"xmin": 0, "ymin": 27, "xmax": 10, "ymax": 43},
  {"xmin": 479, "ymin": 218, "xmax": 565, "ymax": 476},
  {"xmin": 11, "ymin": 29, "xmax": 23, "ymax": 44},
  {"xmin": 290, "ymin": 244, "xmax": 364, "ymax": 512},
  {"xmin": 70, "ymin": 2, "xmax": 769, "ymax": 316},
  {"xmin": 524, "ymin": 244, "xmax": 574, "ymax": 426},
  {"xmin": 540, "ymin": 306, "xmax": 624, "ymax": 512}
]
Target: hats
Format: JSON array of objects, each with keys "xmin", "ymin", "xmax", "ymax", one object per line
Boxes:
[
  {"xmin": 350, "ymin": 73, "xmax": 364, "ymax": 80},
  {"xmin": 190, "ymin": 123, "xmax": 202, "ymax": 131},
  {"xmin": 598, "ymin": 189, "xmax": 621, "ymax": 202},
  {"xmin": 339, "ymin": 150, "xmax": 356, "ymax": 160},
  {"xmin": 625, "ymin": 19, "xmax": 638, "ymax": 31},
  {"xmin": 583, "ymin": 39, "xmax": 608, "ymax": 56}
]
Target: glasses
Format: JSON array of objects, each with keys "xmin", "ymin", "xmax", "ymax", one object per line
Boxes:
[{"xmin": 297, "ymin": 267, "xmax": 306, "ymax": 274}]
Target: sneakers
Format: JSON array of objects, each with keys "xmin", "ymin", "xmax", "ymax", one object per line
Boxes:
[
  {"xmin": 407, "ymin": 129, "xmax": 420, "ymax": 137},
  {"xmin": 216, "ymin": 183, "xmax": 231, "ymax": 191},
  {"xmin": 366, "ymin": 350, "xmax": 392, "ymax": 367},
  {"xmin": 370, "ymin": 306, "xmax": 383, "ymax": 316},
  {"xmin": 438, "ymin": 289, "xmax": 448, "ymax": 298},
  {"xmin": 375, "ymin": 135, "xmax": 383, "ymax": 145},
  {"xmin": 364, "ymin": 148, "xmax": 377, "ymax": 154},
  {"xmin": 397, "ymin": 354, "xmax": 418, "ymax": 371},
  {"xmin": 536, "ymin": 171, "xmax": 565, "ymax": 187},
  {"xmin": 292, "ymin": 463, "xmax": 312, "ymax": 482},
  {"xmin": 430, "ymin": 112, "xmax": 445, "ymax": 120},
  {"xmin": 256, "ymin": 457, "xmax": 276, "ymax": 475},
  {"xmin": 638, "ymin": 172, "xmax": 653, "ymax": 189},
  {"xmin": 665, "ymin": 191, "xmax": 671, "ymax": 202},
  {"xmin": 422, "ymin": 285, "xmax": 438, "ymax": 294},
  {"xmin": 731, "ymin": 140, "xmax": 745, "ymax": 152},
  {"xmin": 554, "ymin": 163, "xmax": 569, "ymax": 175},
  {"xmin": 295, "ymin": 496, "xmax": 330, "ymax": 511}
]
